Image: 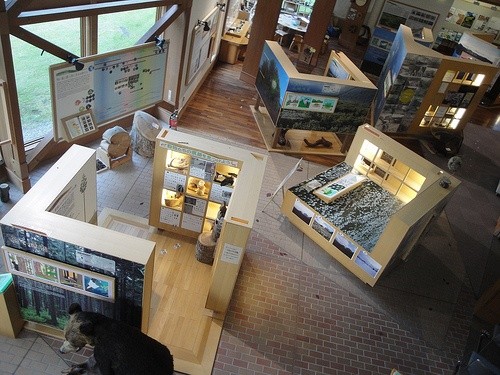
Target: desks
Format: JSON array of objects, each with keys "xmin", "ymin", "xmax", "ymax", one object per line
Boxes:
[{"xmin": 275, "ymin": 13, "xmax": 310, "ymax": 39}]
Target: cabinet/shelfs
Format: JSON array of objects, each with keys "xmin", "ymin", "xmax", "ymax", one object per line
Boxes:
[{"xmin": 218, "ymin": 1, "xmax": 256, "ymax": 65}]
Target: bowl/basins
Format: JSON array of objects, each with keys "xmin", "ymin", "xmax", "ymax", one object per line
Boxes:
[{"xmin": 165, "ymin": 199, "xmax": 180, "ymax": 207}]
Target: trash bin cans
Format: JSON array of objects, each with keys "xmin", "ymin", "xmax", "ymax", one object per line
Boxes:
[
  {"xmin": 443, "ymin": 129, "xmax": 464, "ymax": 156},
  {"xmin": 0, "ymin": 183, "xmax": 10, "ymax": 202}
]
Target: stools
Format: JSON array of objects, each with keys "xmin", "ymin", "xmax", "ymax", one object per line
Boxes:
[{"xmin": 273, "ymin": 30, "xmax": 289, "ymax": 47}]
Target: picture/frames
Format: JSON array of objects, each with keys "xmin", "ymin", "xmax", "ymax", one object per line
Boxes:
[
  {"xmin": 313, "ymin": 172, "xmax": 368, "ymax": 200},
  {"xmin": 281, "ymin": 91, "xmax": 339, "ymax": 114},
  {"xmin": 0, "ymin": 245, "xmax": 116, "ymax": 305}
]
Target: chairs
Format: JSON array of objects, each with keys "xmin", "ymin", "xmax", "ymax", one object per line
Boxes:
[{"xmin": 288, "ymin": 34, "xmax": 304, "ymax": 53}]
